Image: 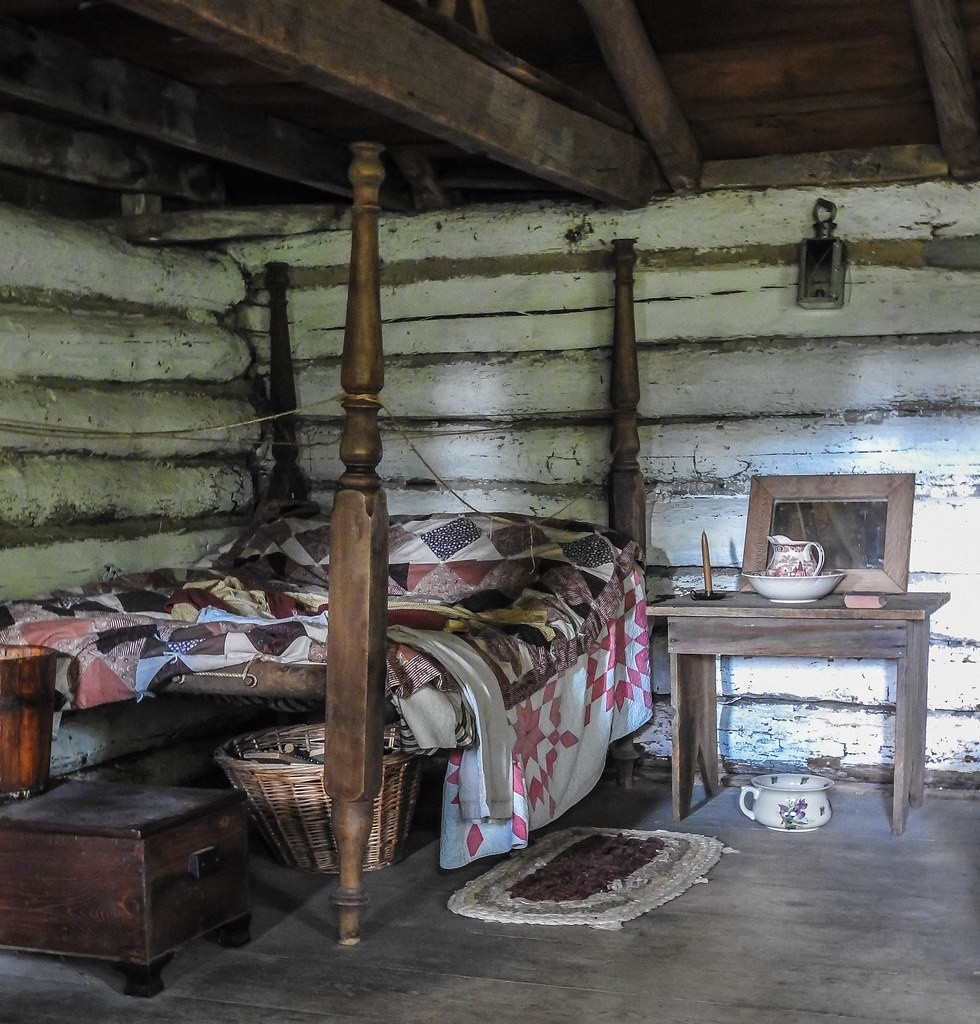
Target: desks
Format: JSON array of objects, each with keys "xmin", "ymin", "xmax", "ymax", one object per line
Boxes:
[{"xmin": 644, "ymin": 590, "xmax": 950, "ymax": 837}]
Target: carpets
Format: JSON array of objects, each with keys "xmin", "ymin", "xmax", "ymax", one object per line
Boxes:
[{"xmin": 447, "ymin": 828, "xmax": 724, "ymax": 932}]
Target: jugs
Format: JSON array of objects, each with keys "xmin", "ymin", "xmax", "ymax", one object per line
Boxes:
[{"xmin": 765, "ymin": 534, "xmax": 825, "ymax": 577}]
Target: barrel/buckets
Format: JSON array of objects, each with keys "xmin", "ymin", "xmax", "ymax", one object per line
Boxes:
[{"xmin": 0, "ymin": 644, "xmax": 59, "ymax": 802}]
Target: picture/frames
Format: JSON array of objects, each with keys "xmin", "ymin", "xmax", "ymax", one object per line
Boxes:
[{"xmin": 739, "ymin": 473, "xmax": 915, "ymax": 595}]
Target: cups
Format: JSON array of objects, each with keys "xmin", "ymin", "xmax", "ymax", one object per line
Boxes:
[{"xmin": 739, "ymin": 772, "xmax": 835, "ymax": 832}]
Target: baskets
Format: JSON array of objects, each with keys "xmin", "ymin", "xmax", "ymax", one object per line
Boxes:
[{"xmin": 213, "ymin": 721, "xmax": 423, "ymax": 875}]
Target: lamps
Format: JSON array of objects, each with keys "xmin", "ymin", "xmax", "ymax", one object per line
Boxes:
[{"xmin": 796, "ymin": 197, "xmax": 847, "ymax": 311}]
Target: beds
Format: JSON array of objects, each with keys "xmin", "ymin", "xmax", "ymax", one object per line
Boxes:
[{"xmin": 1, "ymin": 502, "xmax": 652, "ymax": 944}]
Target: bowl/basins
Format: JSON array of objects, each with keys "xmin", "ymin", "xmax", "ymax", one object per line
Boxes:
[{"xmin": 740, "ymin": 570, "xmax": 847, "ymax": 603}]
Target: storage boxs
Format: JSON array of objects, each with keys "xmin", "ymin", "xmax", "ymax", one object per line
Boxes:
[{"xmin": 0, "ymin": 785, "xmax": 253, "ymax": 998}]
